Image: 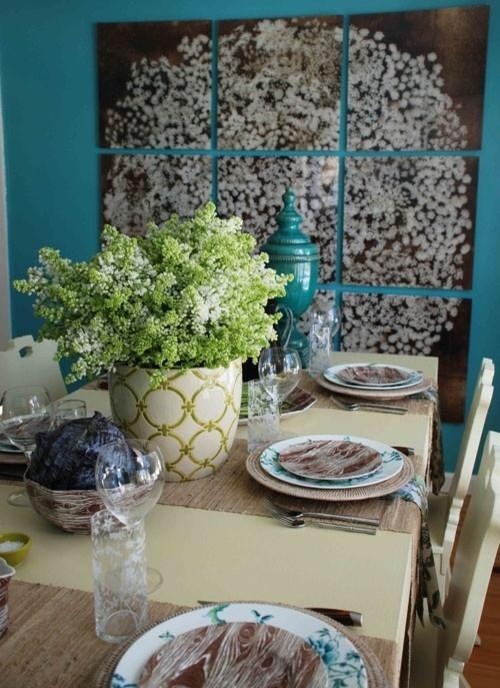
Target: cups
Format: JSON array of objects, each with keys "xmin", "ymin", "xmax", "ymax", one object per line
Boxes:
[
  {"xmin": 308, "ymin": 322, "xmax": 332, "ymax": 376},
  {"xmin": 0, "ymin": 557, "xmax": 16, "ymax": 642},
  {"xmin": 90, "ymin": 507, "xmax": 149, "ymax": 644},
  {"xmin": 57, "ymin": 399, "xmax": 88, "ymax": 424},
  {"xmin": 246, "ymin": 378, "xmax": 281, "ymax": 455}
]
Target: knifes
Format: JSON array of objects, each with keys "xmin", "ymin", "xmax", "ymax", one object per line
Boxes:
[{"xmin": 196, "ymin": 600, "xmax": 364, "ymax": 629}]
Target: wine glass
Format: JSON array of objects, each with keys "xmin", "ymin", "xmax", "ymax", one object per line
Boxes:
[
  {"xmin": 94, "ymin": 441, "xmax": 167, "ymax": 599},
  {"xmin": 0, "ymin": 384, "xmax": 57, "ymax": 508},
  {"xmin": 306, "ymin": 300, "xmax": 342, "ymax": 370},
  {"xmin": 256, "ymin": 346, "xmax": 304, "ymax": 442}
]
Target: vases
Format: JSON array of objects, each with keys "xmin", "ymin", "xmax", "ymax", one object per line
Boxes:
[{"xmin": 108, "ymin": 357, "xmax": 242, "ymax": 482}]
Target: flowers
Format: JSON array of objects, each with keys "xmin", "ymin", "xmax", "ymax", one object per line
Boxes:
[{"xmin": 11, "ymin": 202, "xmax": 295, "ymax": 392}]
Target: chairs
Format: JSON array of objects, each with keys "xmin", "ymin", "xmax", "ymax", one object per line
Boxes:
[
  {"xmin": 0, "ymin": 333, "xmax": 68, "ymax": 417},
  {"xmin": 417, "ymin": 358, "xmax": 500, "ymax": 647},
  {"xmin": 408, "ymin": 431, "xmax": 500, "ymax": 688}
]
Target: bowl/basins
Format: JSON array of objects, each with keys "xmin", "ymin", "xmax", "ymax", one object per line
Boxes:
[{"xmin": 0, "ymin": 532, "xmax": 34, "ymax": 567}]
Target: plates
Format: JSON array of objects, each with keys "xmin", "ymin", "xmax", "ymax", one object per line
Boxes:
[
  {"xmin": 244, "ymin": 432, "xmax": 416, "ymax": 504},
  {"xmin": 0, "ymin": 412, "xmax": 58, "ymax": 455},
  {"xmin": 314, "ymin": 362, "xmax": 435, "ymax": 403},
  {"xmin": 239, "ymin": 374, "xmax": 320, "ymax": 426},
  {"xmin": 87, "ymin": 599, "xmax": 391, "ymax": 688}
]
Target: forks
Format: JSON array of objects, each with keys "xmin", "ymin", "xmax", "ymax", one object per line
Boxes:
[
  {"xmin": 328, "ymin": 395, "xmax": 409, "ymax": 416},
  {"xmin": 268, "ymin": 511, "xmax": 377, "ymax": 535},
  {"xmin": 263, "ymin": 497, "xmax": 380, "ymax": 526}
]
